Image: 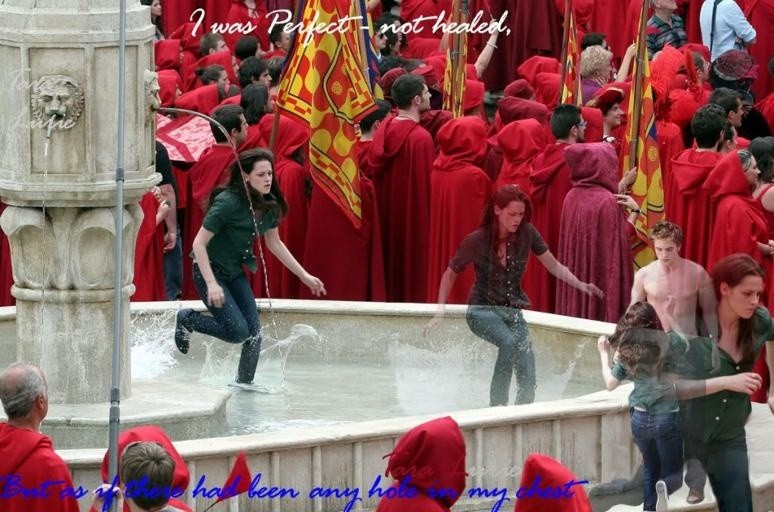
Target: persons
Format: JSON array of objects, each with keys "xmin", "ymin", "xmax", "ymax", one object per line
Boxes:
[{"xmin": 2, "ymin": 0, "xmax": 773, "ymax": 512}]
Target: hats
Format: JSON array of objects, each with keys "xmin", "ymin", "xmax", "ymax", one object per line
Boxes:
[{"xmin": 586, "ymin": 87, "xmax": 625, "ymax": 117}]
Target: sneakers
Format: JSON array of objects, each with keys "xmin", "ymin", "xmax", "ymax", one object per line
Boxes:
[{"xmin": 174, "ymin": 308, "xmax": 195, "ymax": 354}]
